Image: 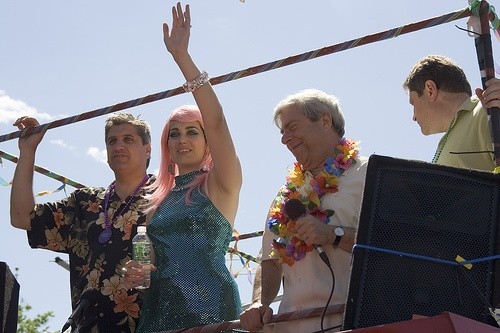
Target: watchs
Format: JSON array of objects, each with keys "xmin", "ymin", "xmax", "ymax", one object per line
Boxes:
[{"xmin": 333, "ymin": 226, "xmax": 345, "ymax": 249}]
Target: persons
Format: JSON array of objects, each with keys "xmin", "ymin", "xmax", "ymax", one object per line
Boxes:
[
  {"xmin": 122, "ymin": 2, "xmax": 243, "ymax": 333},
  {"xmin": 403, "ymin": 55, "xmax": 500, "ymax": 172},
  {"xmin": 239, "ymin": 88, "xmax": 369, "ymax": 333},
  {"xmin": 10, "ymin": 112, "xmax": 158, "ymax": 333}
]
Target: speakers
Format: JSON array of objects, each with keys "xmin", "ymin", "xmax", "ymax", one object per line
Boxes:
[{"xmin": 341, "ymin": 155, "xmax": 500, "ymax": 330}]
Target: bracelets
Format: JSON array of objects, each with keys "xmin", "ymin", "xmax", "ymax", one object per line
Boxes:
[{"xmin": 183, "ymin": 70, "xmax": 210, "ymax": 93}]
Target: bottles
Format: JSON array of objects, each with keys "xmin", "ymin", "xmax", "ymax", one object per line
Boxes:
[{"xmin": 132, "ymin": 226, "xmax": 150, "ymax": 289}]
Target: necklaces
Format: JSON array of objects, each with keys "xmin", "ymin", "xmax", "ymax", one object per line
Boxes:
[
  {"xmin": 98, "ymin": 175, "xmax": 149, "ymax": 243},
  {"xmin": 268, "ymin": 136, "xmax": 360, "ymax": 266}
]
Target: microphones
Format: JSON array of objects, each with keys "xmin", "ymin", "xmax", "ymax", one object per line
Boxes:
[{"xmin": 286, "ymin": 198, "xmax": 330, "ymax": 267}]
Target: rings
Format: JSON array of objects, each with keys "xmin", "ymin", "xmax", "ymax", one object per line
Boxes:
[{"xmin": 185, "ymin": 25, "xmax": 191, "ymax": 28}]
